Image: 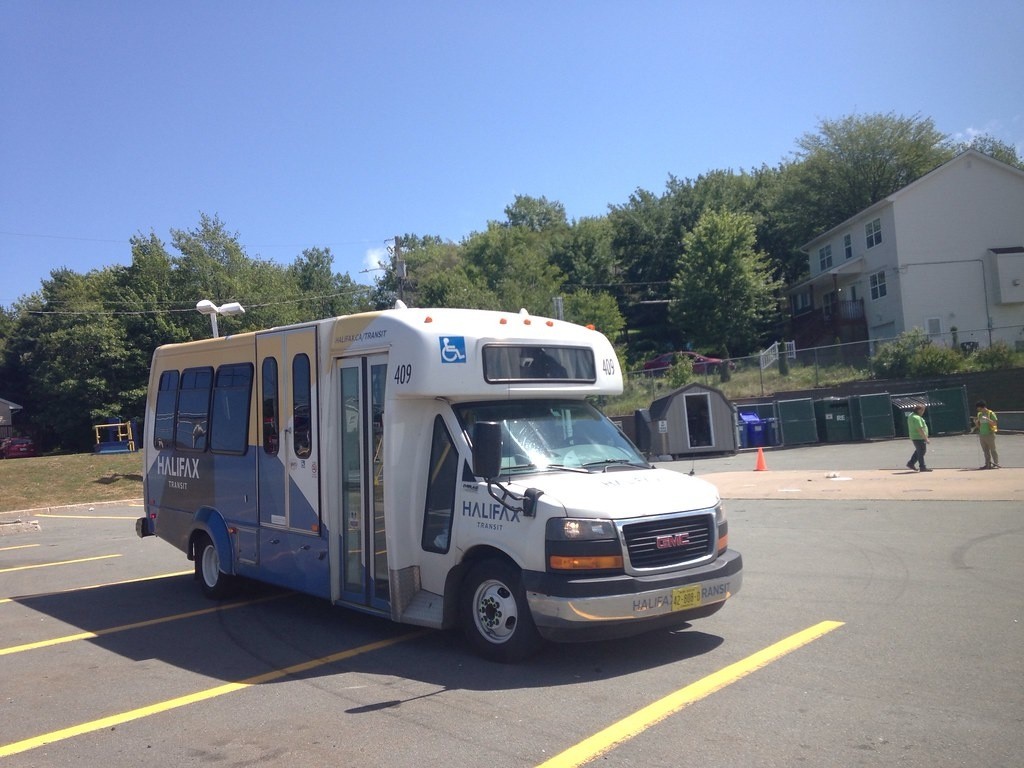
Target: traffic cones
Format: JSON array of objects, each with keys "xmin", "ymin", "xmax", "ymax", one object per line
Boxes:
[{"xmin": 752, "ymin": 448, "xmax": 769, "ymax": 471}]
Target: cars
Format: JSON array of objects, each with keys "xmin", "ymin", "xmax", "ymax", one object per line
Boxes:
[
  {"xmin": 0, "ymin": 435, "xmax": 43, "ymax": 459},
  {"xmin": 644, "ymin": 351, "xmax": 737, "ymax": 380}
]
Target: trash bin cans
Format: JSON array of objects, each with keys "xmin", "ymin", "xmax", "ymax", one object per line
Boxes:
[{"xmin": 737, "ymin": 412, "xmax": 779, "ymax": 449}]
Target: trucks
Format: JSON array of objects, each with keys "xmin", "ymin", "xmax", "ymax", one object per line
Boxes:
[{"xmin": 135, "ymin": 298, "xmax": 746, "ymax": 666}]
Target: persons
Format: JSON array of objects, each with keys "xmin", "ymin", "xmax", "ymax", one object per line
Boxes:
[
  {"xmin": 906, "ymin": 404, "xmax": 934, "ymax": 472},
  {"xmin": 974, "ymin": 400, "xmax": 1002, "ymax": 469}
]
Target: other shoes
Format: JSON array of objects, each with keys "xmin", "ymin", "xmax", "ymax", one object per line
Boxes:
[
  {"xmin": 907, "ymin": 463, "xmax": 918, "ymax": 471},
  {"xmin": 920, "ymin": 468, "xmax": 933, "ymax": 472},
  {"xmin": 980, "ymin": 465, "xmax": 991, "ymax": 469},
  {"xmin": 992, "ymin": 463, "xmax": 999, "ymax": 469}
]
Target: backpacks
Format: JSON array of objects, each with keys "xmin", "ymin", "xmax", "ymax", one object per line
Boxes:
[{"xmin": 978, "ymin": 410, "xmax": 997, "ymax": 432}]
